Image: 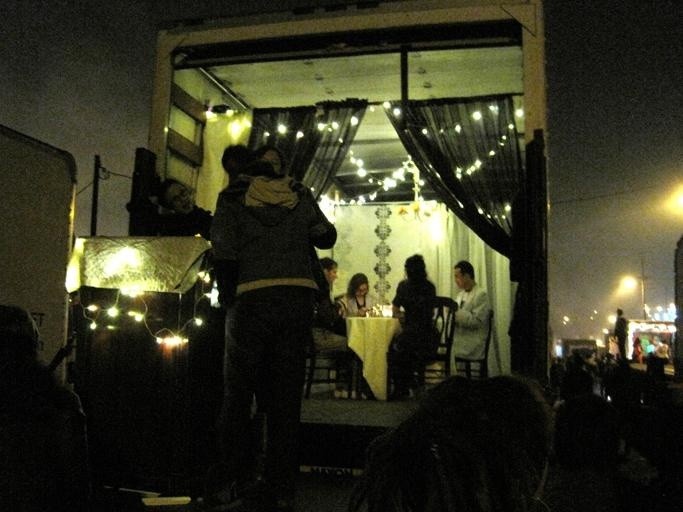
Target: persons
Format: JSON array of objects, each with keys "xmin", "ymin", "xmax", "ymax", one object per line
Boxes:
[{"xmin": 0, "ymin": 144, "xmax": 683, "ymax": 512}]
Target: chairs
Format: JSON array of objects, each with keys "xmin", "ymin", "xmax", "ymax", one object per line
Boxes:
[
  {"xmin": 303, "ymin": 336, "xmax": 358, "ymax": 401},
  {"xmin": 455, "ymin": 309, "xmax": 494, "ymax": 380},
  {"xmin": 386, "ymin": 296, "xmax": 459, "ymax": 402}
]
